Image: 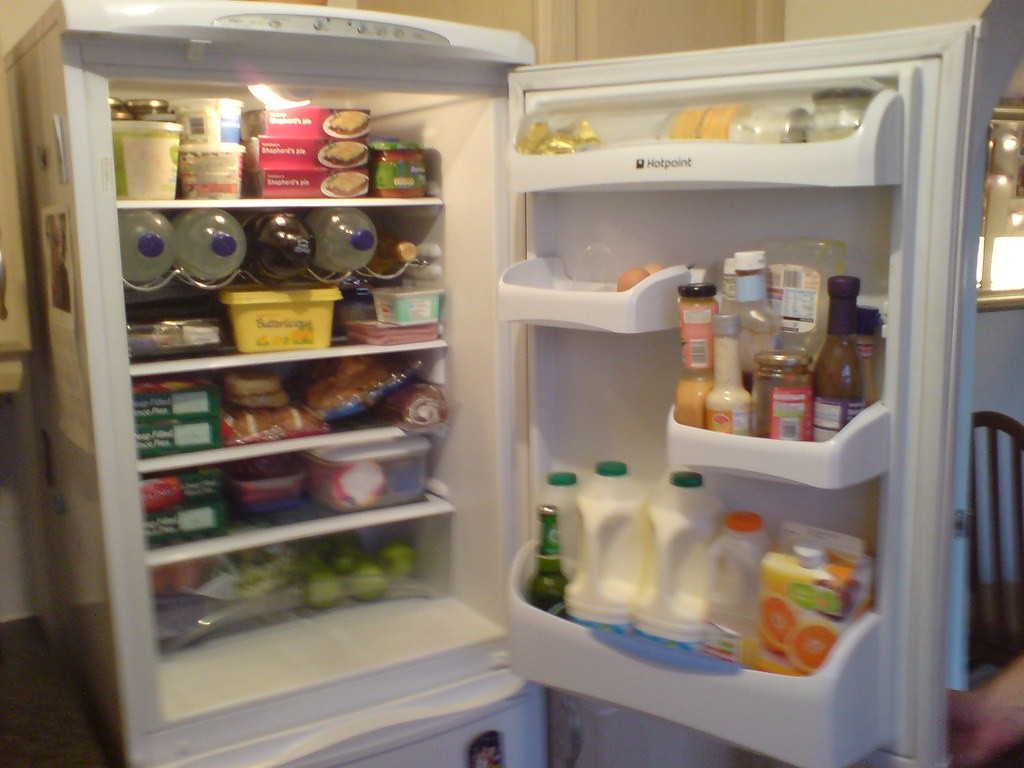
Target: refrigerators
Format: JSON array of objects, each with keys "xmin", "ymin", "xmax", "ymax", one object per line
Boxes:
[{"xmin": 0, "ymin": 0, "xmax": 991, "ymax": 768}]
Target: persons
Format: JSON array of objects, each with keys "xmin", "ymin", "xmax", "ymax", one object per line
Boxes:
[{"xmin": 947, "ymin": 653, "xmax": 1024, "ymax": 768}]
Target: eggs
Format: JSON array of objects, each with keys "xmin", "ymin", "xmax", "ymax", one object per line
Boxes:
[{"xmin": 615, "ymin": 263, "xmax": 663, "ymax": 291}]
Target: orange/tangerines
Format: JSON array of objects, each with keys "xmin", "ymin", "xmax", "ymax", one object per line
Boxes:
[{"xmin": 756, "ymin": 593, "xmax": 839, "ymax": 671}]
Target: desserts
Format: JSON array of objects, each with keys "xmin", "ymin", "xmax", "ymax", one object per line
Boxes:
[{"xmin": 322, "ymin": 111, "xmax": 369, "ymax": 195}]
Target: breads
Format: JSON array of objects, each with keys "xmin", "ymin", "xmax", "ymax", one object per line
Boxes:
[{"xmin": 216, "ymin": 356, "xmax": 387, "ymax": 432}]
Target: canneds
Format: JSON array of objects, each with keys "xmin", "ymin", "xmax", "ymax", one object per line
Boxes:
[
  {"xmin": 370, "ymin": 139, "xmax": 426, "ymax": 199},
  {"xmin": 750, "ymin": 351, "xmax": 817, "ymax": 441}
]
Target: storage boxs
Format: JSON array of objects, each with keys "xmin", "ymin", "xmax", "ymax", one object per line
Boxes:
[
  {"xmin": 130, "ymin": 375, "xmax": 431, "ymax": 543},
  {"xmin": 219, "ymin": 283, "xmax": 345, "ymax": 351},
  {"xmin": 240, "ymin": 106, "xmax": 372, "ymax": 199}
]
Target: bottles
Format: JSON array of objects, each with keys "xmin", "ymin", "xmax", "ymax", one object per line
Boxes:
[
  {"xmin": 706, "ymin": 313, "xmax": 752, "ymax": 436},
  {"xmin": 527, "ymin": 503, "xmax": 567, "ymax": 620},
  {"xmin": 701, "ymin": 512, "xmax": 771, "ymax": 658},
  {"xmin": 369, "ymin": 229, "xmax": 418, "ymax": 278},
  {"xmin": 630, "ymin": 468, "xmax": 725, "ymax": 645},
  {"xmin": 658, "ymin": 106, "xmax": 813, "ymax": 144},
  {"xmin": 809, "ymin": 274, "xmax": 861, "ymax": 441},
  {"xmin": 116, "ymin": 210, "xmax": 179, "ymax": 282},
  {"xmin": 240, "ymin": 210, "xmax": 317, "ymax": 280},
  {"xmin": 171, "ymin": 210, "xmax": 248, "ymax": 282},
  {"xmin": 715, "ymin": 259, "xmax": 738, "ymax": 339},
  {"xmin": 736, "ymin": 250, "xmax": 781, "ymax": 396},
  {"xmin": 526, "ymin": 474, "xmax": 581, "ymax": 589},
  {"xmin": 564, "ymin": 459, "xmax": 647, "ymax": 628},
  {"xmin": 305, "ymin": 208, "xmax": 378, "ymax": 276},
  {"xmin": 847, "ymin": 307, "xmax": 881, "ymax": 420}
]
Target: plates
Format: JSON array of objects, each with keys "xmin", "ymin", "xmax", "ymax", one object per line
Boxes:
[
  {"xmin": 318, "ymin": 141, "xmax": 369, "ymax": 170},
  {"xmin": 323, "ymin": 111, "xmax": 371, "ymax": 138},
  {"xmin": 321, "ymin": 172, "xmax": 368, "ymax": 199}
]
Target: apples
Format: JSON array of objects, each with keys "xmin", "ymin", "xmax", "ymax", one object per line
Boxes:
[{"xmin": 299, "ymin": 535, "xmax": 414, "ymax": 606}]
